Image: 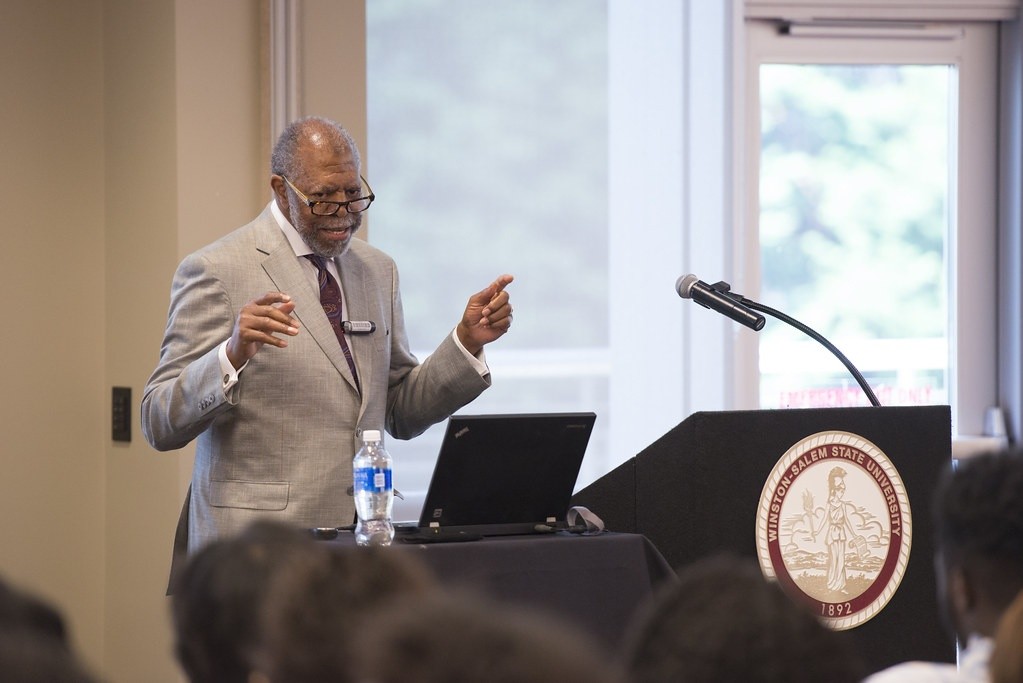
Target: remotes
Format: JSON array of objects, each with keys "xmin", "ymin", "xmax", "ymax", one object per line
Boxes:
[{"xmin": 306, "ymin": 527, "xmax": 338, "ymax": 540}]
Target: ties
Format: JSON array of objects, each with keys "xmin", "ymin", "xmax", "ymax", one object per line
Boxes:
[{"xmin": 305, "ymin": 254, "xmax": 361, "ymax": 397}]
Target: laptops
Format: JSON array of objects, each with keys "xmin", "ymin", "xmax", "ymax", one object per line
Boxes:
[{"xmin": 349, "ymin": 412, "xmax": 597, "ymax": 542}]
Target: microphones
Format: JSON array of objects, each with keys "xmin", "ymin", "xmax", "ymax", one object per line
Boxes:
[{"xmin": 675, "ymin": 274, "xmax": 766, "ymax": 331}]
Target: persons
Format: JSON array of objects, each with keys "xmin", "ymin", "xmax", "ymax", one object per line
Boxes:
[
  {"xmin": 1, "ymin": 448, "xmax": 1023, "ymax": 683},
  {"xmin": 141, "ymin": 119, "xmax": 516, "ymax": 592}
]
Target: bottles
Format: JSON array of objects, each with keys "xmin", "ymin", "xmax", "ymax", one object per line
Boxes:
[{"xmin": 352, "ymin": 430, "xmax": 395, "ymax": 547}]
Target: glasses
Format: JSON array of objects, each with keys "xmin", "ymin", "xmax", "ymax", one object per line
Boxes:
[{"xmin": 282, "ymin": 173, "xmax": 375, "ymax": 217}]
